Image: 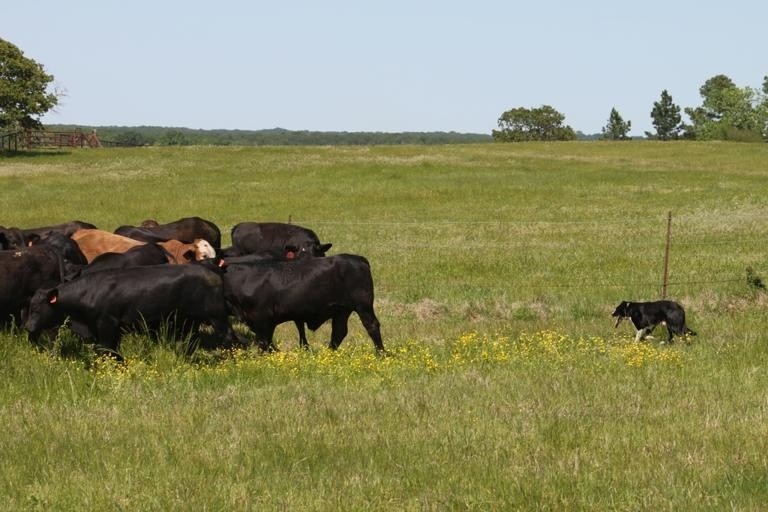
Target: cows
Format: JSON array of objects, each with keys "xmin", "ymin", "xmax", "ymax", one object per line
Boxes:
[{"xmin": 0, "ymin": 215, "xmax": 386, "ymax": 362}]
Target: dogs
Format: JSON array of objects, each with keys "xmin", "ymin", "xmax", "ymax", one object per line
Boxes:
[{"xmin": 610, "ymin": 300, "xmax": 697, "ymax": 344}]
